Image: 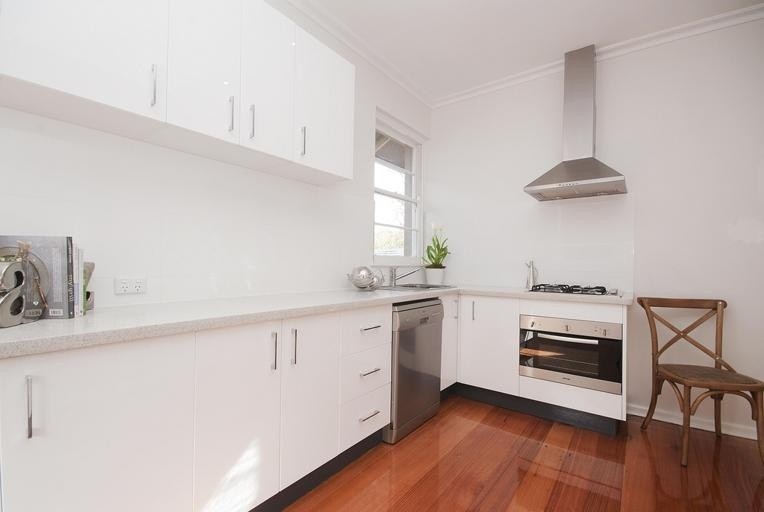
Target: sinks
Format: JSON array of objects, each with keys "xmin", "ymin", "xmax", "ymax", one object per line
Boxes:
[{"xmin": 382, "ymin": 284, "xmax": 447, "ymax": 289}]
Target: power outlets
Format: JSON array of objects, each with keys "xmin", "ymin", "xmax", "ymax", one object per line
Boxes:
[{"xmin": 114, "ymin": 278, "xmax": 148, "ymax": 294}]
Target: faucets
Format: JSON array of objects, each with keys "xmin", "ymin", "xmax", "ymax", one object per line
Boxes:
[{"xmin": 390, "ymin": 265, "xmax": 428, "ymax": 285}]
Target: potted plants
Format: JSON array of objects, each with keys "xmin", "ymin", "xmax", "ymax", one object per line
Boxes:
[{"xmin": 420, "ymin": 234, "xmax": 452, "ymax": 285}]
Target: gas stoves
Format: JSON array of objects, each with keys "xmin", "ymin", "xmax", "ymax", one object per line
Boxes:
[{"xmin": 529, "ymin": 284, "xmax": 609, "ymax": 296}]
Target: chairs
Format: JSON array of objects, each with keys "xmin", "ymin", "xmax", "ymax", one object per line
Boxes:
[{"xmin": 636, "ymin": 296, "xmax": 762, "ymax": 468}]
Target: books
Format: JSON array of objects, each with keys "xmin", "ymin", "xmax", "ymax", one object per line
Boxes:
[{"xmin": 36, "ymin": 234, "xmax": 87, "ymax": 318}]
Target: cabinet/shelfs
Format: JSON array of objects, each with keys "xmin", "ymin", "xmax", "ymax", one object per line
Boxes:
[
  {"xmin": 1, "ymin": 1, "xmax": 166, "ymax": 139},
  {"xmin": 441, "ymin": 290, "xmax": 459, "ymax": 411},
  {"xmin": 166, "ymin": 0, "xmax": 292, "ymax": 172},
  {"xmin": 0, "ymin": 339, "xmax": 195, "ymax": 512},
  {"xmin": 293, "ymin": 26, "xmax": 354, "ymax": 187},
  {"xmin": 194, "ymin": 314, "xmax": 342, "ymax": 512},
  {"xmin": 341, "ymin": 303, "xmax": 392, "ymax": 461},
  {"xmin": 459, "ymin": 295, "xmax": 519, "ymax": 410}
]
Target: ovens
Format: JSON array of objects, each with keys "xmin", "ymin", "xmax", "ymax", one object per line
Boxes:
[{"xmin": 519, "ymin": 314, "xmax": 623, "ymax": 395}]
